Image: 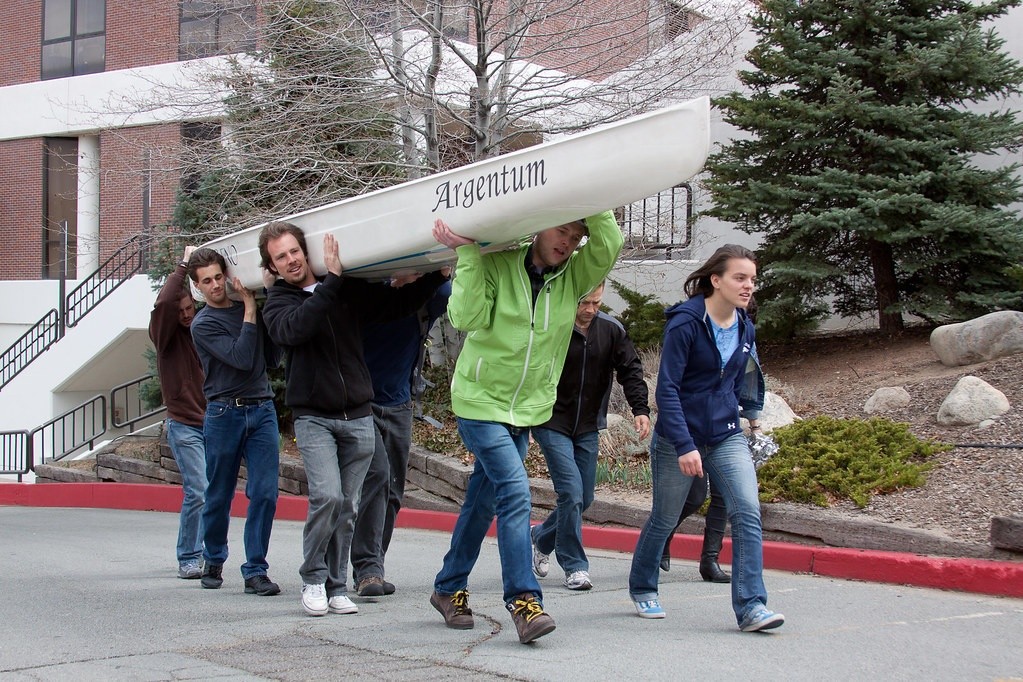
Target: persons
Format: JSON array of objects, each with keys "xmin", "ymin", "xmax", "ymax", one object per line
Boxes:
[
  {"xmin": 529, "ymin": 278, "xmax": 650, "ymax": 590},
  {"xmin": 430, "ymin": 207, "xmax": 624, "ymax": 644},
  {"xmin": 257, "ymin": 220, "xmax": 454, "ymax": 617},
  {"xmin": 149, "ymin": 245, "xmax": 209, "ymax": 579},
  {"xmin": 628, "ymin": 244, "xmax": 785, "ymax": 633},
  {"xmin": 187, "ymin": 248, "xmax": 284, "ymax": 595}
]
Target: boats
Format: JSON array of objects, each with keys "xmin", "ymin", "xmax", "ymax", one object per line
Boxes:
[{"xmin": 186, "ymin": 94, "xmax": 712, "ymax": 301}]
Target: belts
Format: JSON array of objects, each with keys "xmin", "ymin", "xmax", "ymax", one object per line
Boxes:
[{"xmin": 212, "ymin": 396, "xmax": 266, "ymax": 407}]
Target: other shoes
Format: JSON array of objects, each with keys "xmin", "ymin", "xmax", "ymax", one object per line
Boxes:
[
  {"xmin": 354, "ymin": 577, "xmax": 395, "ymax": 597},
  {"xmin": 178, "ymin": 562, "xmax": 202, "ymax": 580}
]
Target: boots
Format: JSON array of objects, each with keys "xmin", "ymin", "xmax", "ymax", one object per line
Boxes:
[
  {"xmin": 698, "ymin": 527, "xmax": 732, "ymax": 583},
  {"xmin": 659, "ymin": 517, "xmax": 685, "ymax": 572}
]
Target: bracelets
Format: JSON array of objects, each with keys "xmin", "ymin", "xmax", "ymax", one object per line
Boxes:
[{"xmin": 748, "ymin": 425, "xmax": 760, "ymax": 430}]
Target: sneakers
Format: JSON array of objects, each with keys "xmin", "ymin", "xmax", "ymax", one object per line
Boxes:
[
  {"xmin": 530, "ymin": 525, "xmax": 550, "ymax": 577},
  {"xmin": 630, "ymin": 591, "xmax": 665, "ymax": 619},
  {"xmin": 430, "ymin": 591, "xmax": 475, "ymax": 629},
  {"xmin": 741, "ymin": 602, "xmax": 785, "ymax": 633},
  {"xmin": 244, "ymin": 575, "xmax": 281, "ymax": 596},
  {"xmin": 301, "ymin": 580, "xmax": 359, "ymax": 616},
  {"xmin": 201, "ymin": 562, "xmax": 223, "ymax": 590},
  {"xmin": 508, "ymin": 593, "xmax": 558, "ymax": 644},
  {"xmin": 563, "ymin": 572, "xmax": 593, "ymax": 591}
]
